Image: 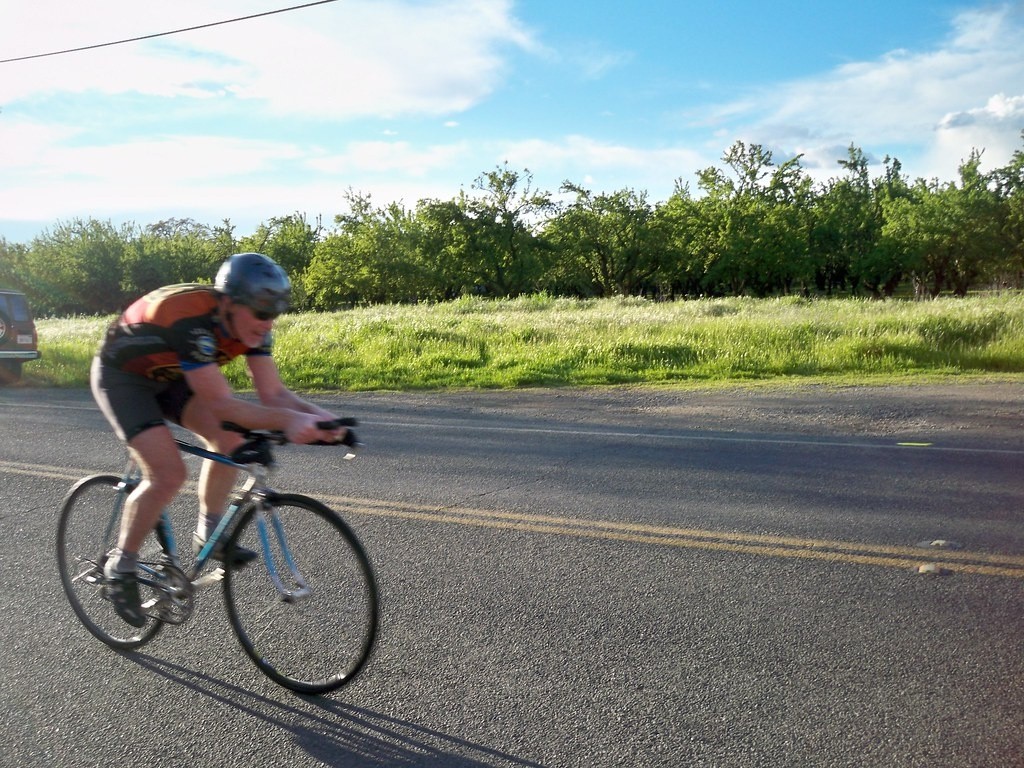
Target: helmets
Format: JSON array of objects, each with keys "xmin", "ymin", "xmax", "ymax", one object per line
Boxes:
[{"xmin": 214, "ymin": 252, "xmax": 291, "ymax": 315}]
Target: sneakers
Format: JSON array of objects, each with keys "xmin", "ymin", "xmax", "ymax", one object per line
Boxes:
[
  {"xmin": 103, "ymin": 557, "xmax": 145, "ymax": 628},
  {"xmin": 191, "ymin": 530, "xmax": 258, "ymax": 565}
]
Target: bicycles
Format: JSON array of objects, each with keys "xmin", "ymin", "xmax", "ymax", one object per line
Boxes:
[{"xmin": 54, "ymin": 414, "xmax": 379, "ymax": 694}]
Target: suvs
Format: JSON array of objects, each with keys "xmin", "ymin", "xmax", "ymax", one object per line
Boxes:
[{"xmin": 1, "ymin": 290, "xmax": 43, "ymax": 382}]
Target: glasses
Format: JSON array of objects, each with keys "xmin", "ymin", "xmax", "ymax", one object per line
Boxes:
[{"xmin": 230, "ymin": 297, "xmax": 280, "ymax": 321}]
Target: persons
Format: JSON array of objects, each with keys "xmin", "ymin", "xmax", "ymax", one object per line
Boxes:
[{"xmin": 90, "ymin": 254, "xmax": 348, "ymax": 628}]
